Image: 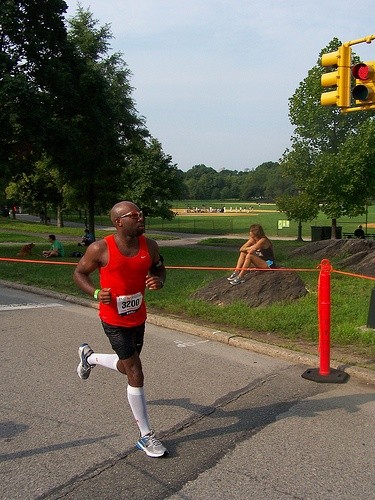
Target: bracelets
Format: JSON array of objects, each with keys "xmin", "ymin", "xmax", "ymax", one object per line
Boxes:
[
  {"xmin": 94, "ymin": 288, "xmax": 100, "ymax": 301},
  {"xmin": 160, "ymin": 280, "xmax": 164, "ymax": 288}
]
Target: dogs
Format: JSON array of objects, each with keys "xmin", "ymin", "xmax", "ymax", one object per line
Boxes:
[
  {"xmin": 66, "ymin": 251, "xmax": 84, "ymax": 259},
  {"xmin": 77, "ymin": 242, "xmax": 83, "ymax": 247},
  {"xmin": 17, "ymin": 242, "xmax": 40, "ymax": 257}
]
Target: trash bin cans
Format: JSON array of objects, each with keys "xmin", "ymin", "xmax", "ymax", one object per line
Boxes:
[
  {"xmin": 310, "ymin": 226, "xmax": 322, "ymax": 242},
  {"xmin": 9, "ymin": 210, "xmax": 16, "ymax": 220},
  {"xmin": 321, "ymin": 225, "xmax": 342, "ymax": 240}
]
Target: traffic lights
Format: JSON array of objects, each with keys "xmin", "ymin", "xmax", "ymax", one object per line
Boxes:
[
  {"xmin": 320, "ymin": 45, "xmax": 351, "ymax": 108},
  {"xmin": 350, "ymin": 62, "xmax": 375, "ymax": 105}
]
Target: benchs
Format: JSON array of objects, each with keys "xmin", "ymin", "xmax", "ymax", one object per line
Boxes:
[{"xmin": 342, "ymin": 232, "xmax": 375, "ymax": 240}]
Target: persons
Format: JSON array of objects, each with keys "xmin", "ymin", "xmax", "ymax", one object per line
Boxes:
[
  {"xmin": 226, "ymin": 224, "xmax": 275, "ymax": 286},
  {"xmin": 40, "ymin": 235, "xmax": 65, "ymax": 258},
  {"xmin": 78, "ymin": 229, "xmax": 94, "ymax": 247},
  {"xmin": 76, "ymin": 201, "xmax": 169, "ymax": 458},
  {"xmin": 354, "ymin": 225, "xmax": 365, "ymax": 239}
]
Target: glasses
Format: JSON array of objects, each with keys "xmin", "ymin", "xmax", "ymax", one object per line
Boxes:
[{"xmin": 114, "ymin": 210, "xmax": 144, "ymax": 222}]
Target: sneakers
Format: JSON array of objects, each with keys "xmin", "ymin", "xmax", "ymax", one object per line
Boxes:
[
  {"xmin": 136, "ymin": 429, "xmax": 170, "ymax": 457},
  {"xmin": 230, "ymin": 276, "xmax": 245, "ymax": 285},
  {"xmin": 76, "ymin": 343, "xmax": 97, "ymax": 379},
  {"xmin": 227, "ymin": 271, "xmax": 239, "ymax": 281}
]
